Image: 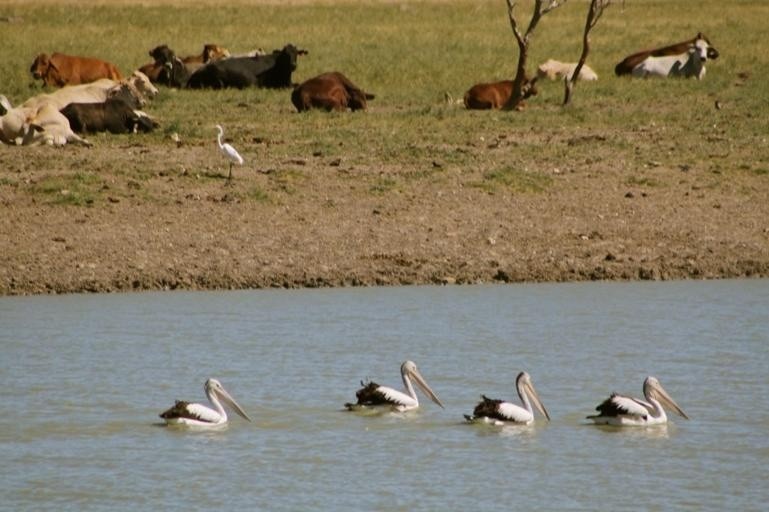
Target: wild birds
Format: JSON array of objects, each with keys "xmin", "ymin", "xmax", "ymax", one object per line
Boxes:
[
  {"xmin": 585, "ymin": 376, "xmax": 690, "ymax": 425},
  {"xmin": 461, "ymin": 370, "xmax": 552, "ymax": 427},
  {"xmin": 212, "ymin": 123, "xmax": 244, "ymax": 180},
  {"xmin": 343, "ymin": 359, "xmax": 444, "ymax": 413},
  {"xmin": 157, "ymin": 377, "xmax": 251, "ymax": 427}
]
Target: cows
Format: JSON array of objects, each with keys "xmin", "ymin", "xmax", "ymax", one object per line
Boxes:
[
  {"xmin": 290, "ymin": 70, "xmax": 375, "ymax": 113},
  {"xmin": 463, "ymin": 75, "xmax": 538, "ymax": 111},
  {"xmin": 0, "ymin": 69, "xmax": 160, "ymax": 150},
  {"xmin": 29, "ymin": 52, "xmax": 124, "ymax": 88},
  {"xmin": 630, "ymin": 39, "xmax": 710, "ymax": 81},
  {"xmin": 614, "ymin": 30, "xmax": 720, "ymax": 76},
  {"xmin": 137, "ymin": 43, "xmax": 309, "ymax": 89},
  {"xmin": 537, "ymin": 59, "xmax": 598, "ymax": 82}
]
